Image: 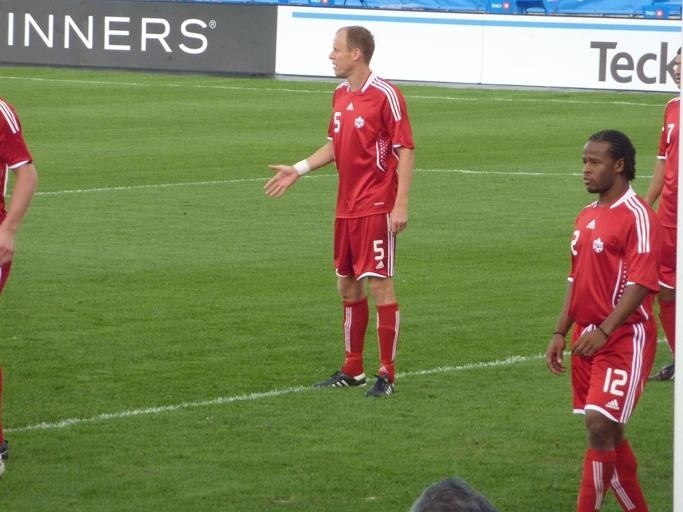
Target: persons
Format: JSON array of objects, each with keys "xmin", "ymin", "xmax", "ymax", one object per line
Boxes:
[
  {"xmin": 641, "ymin": 47, "xmax": 674, "ymax": 383},
  {"xmin": 543, "ymin": 128, "xmax": 662, "ymax": 509},
  {"xmin": 0, "ymin": 94, "xmax": 37, "ymax": 478},
  {"xmin": 262, "ymin": 25, "xmax": 417, "ymax": 399}
]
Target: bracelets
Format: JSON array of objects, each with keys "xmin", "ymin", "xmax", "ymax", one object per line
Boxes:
[
  {"xmin": 596, "ymin": 325, "xmax": 610, "ymax": 341},
  {"xmin": 552, "ymin": 330, "xmax": 567, "ymax": 338},
  {"xmin": 290, "ymin": 158, "xmax": 311, "ymax": 177}
]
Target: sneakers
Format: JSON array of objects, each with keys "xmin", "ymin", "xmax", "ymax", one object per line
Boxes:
[
  {"xmin": 647, "ymin": 363, "xmax": 674, "ymax": 380},
  {"xmin": 365, "ymin": 374, "xmax": 396, "ymax": 397},
  {"xmin": 314, "ymin": 370, "xmax": 368, "ymax": 387}
]
